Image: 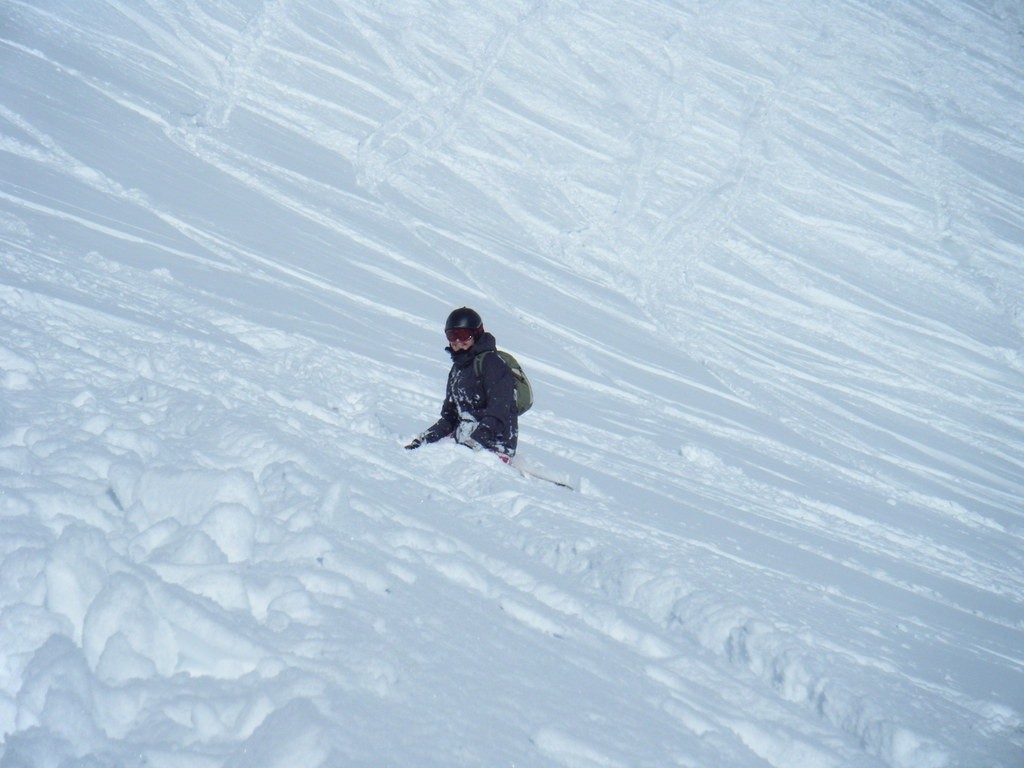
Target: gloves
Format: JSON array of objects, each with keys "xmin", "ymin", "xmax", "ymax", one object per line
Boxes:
[
  {"xmin": 404, "ymin": 438, "xmax": 423, "ymax": 450},
  {"xmin": 461, "ymin": 437, "xmax": 478, "ymax": 449}
]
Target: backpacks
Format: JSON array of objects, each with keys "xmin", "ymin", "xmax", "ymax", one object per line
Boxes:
[{"xmin": 474, "ymin": 351, "xmax": 534, "ymax": 416}]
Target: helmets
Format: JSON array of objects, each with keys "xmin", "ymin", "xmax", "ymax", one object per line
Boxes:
[{"xmin": 444, "ymin": 305, "xmax": 484, "ymax": 338}]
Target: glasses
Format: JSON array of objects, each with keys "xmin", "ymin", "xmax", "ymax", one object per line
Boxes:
[{"xmin": 445, "ymin": 329, "xmax": 475, "ymax": 341}]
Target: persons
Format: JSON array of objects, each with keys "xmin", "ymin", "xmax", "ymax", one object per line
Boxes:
[{"xmin": 404, "ymin": 306, "xmax": 518, "ymax": 466}]
